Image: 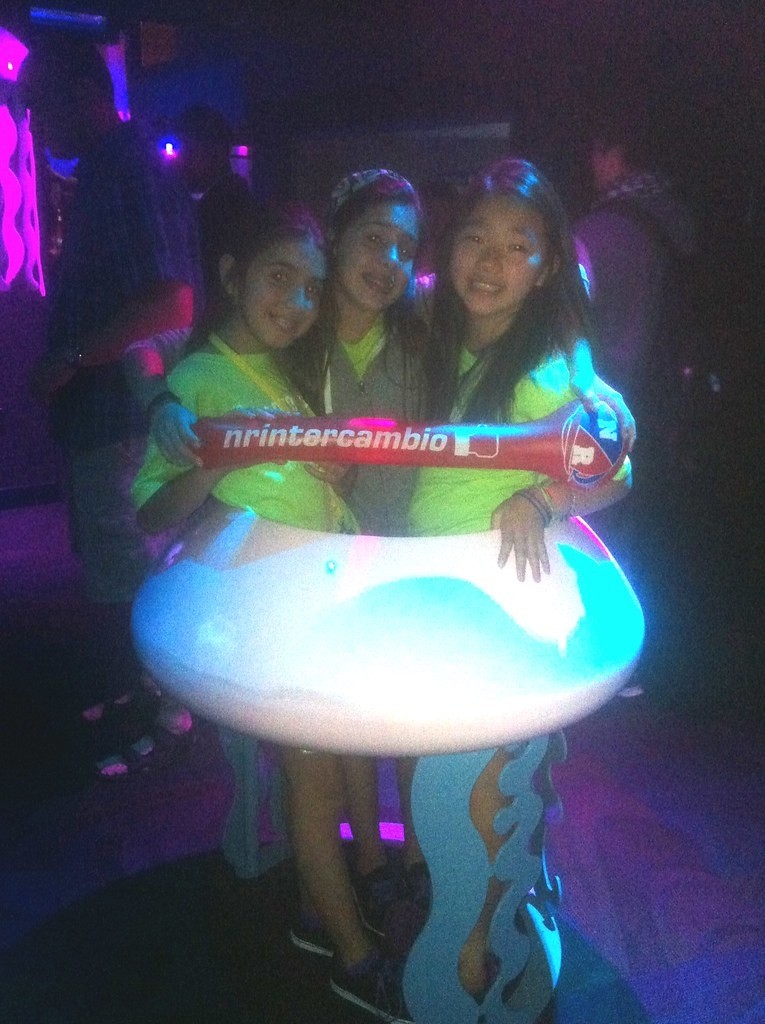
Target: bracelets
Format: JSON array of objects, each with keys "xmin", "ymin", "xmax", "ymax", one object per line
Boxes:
[
  {"xmin": 143, "ymin": 391, "xmax": 181, "ymax": 416},
  {"xmin": 516, "ymin": 485, "xmax": 576, "ymax": 528}
]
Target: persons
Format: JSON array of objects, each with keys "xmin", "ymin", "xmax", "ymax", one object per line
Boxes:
[{"xmin": 0, "ymin": 33, "xmax": 765, "ymax": 1024}]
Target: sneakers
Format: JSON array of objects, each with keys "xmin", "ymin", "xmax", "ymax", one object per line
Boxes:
[
  {"xmin": 353, "ymin": 843, "xmax": 404, "ymax": 935},
  {"xmin": 288, "ymin": 910, "xmax": 335, "ymax": 956},
  {"xmin": 327, "ymin": 936, "xmax": 411, "ymax": 1023}
]
